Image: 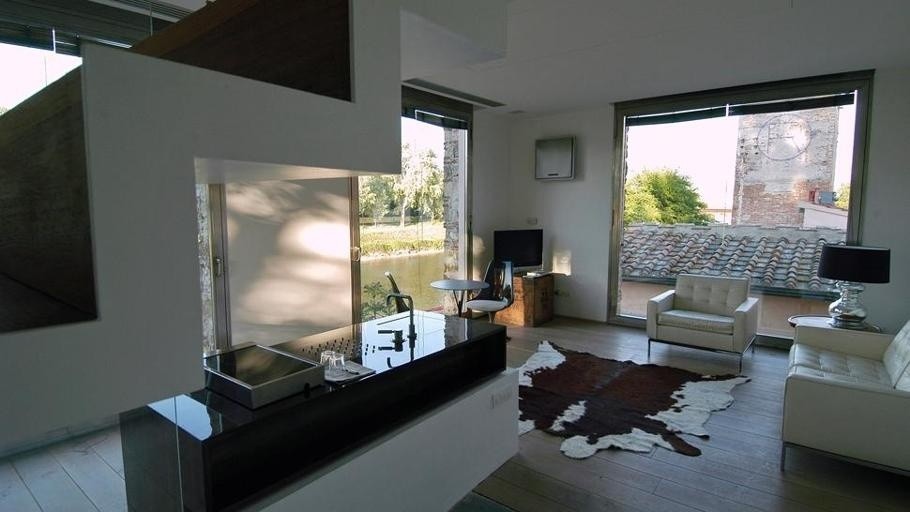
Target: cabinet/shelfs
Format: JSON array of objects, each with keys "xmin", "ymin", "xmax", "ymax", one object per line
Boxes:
[{"xmin": 494, "ymin": 274, "xmax": 555, "ymax": 328}]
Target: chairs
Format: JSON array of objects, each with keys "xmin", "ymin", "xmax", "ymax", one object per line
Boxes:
[
  {"xmin": 385, "ymin": 270, "xmax": 411, "ymax": 312},
  {"xmin": 465, "ymin": 257, "xmax": 514, "ymax": 324}
]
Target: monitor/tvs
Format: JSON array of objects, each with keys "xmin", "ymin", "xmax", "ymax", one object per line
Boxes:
[{"xmin": 492, "ymin": 228, "xmax": 543, "ymax": 274}]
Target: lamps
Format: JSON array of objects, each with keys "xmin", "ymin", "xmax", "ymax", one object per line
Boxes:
[{"xmin": 817, "ymin": 243, "xmax": 891, "ymax": 330}]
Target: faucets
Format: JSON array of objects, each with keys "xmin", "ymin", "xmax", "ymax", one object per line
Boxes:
[
  {"xmin": 386, "ymin": 293, "xmax": 417, "ymax": 349},
  {"xmin": 387, "ymin": 349, "xmax": 416, "ymax": 367}
]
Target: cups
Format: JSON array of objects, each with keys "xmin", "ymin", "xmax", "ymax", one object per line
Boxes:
[{"xmin": 319, "ymin": 349, "xmax": 347, "ymax": 379}]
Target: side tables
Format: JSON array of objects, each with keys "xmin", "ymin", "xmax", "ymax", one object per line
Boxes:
[{"xmin": 787, "ymin": 314, "xmax": 884, "ymax": 333}]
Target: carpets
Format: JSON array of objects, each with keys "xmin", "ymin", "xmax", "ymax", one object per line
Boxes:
[{"xmin": 509, "ymin": 338, "xmax": 752, "ymax": 460}]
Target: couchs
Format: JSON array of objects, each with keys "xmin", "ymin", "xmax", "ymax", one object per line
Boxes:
[
  {"xmin": 646, "ymin": 273, "xmax": 760, "ymax": 374},
  {"xmin": 779, "ymin": 322, "xmax": 910, "ymax": 472}
]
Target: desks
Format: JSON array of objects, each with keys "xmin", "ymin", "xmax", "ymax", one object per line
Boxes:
[{"xmin": 431, "ymin": 279, "xmax": 490, "ymax": 318}]
